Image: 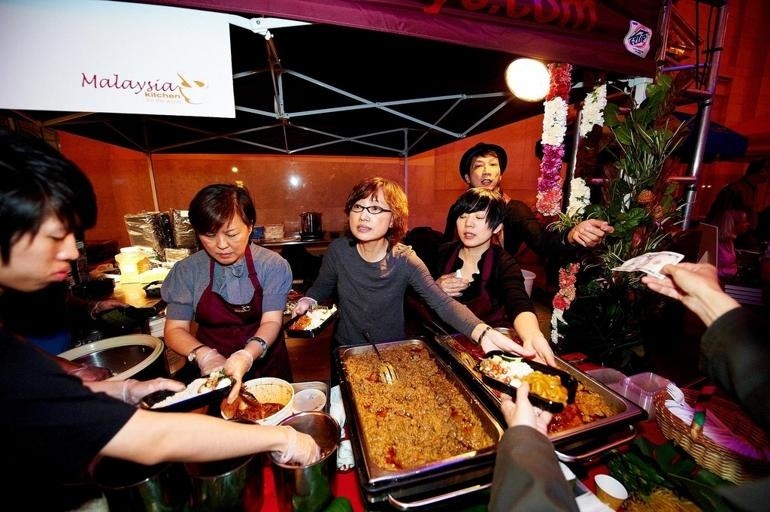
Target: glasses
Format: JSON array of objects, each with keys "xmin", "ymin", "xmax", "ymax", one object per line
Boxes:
[{"xmin": 348, "ymin": 202, "xmax": 392, "ymax": 217}]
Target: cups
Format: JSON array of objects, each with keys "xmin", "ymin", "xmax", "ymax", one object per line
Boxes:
[{"xmin": 595, "ymin": 474, "xmax": 627, "ymax": 511}]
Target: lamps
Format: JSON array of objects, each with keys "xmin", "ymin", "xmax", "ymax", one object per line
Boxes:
[{"xmin": 505, "ymin": 57, "xmax": 551, "ymax": 102}]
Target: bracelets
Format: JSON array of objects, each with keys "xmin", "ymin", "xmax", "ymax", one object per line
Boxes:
[
  {"xmin": 245, "ymin": 336, "xmax": 268, "ymax": 361},
  {"xmin": 476, "ymin": 326, "xmax": 492, "ymax": 344}
]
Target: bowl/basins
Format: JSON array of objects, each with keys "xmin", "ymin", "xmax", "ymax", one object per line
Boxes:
[
  {"xmin": 186, "ymin": 418, "xmax": 260, "ymax": 512},
  {"xmin": 97, "ymin": 468, "xmax": 166, "ymax": 512},
  {"xmin": 221, "ymin": 377, "xmax": 294, "ymax": 426},
  {"xmin": 267, "ymin": 412, "xmax": 341, "ymax": 512}
]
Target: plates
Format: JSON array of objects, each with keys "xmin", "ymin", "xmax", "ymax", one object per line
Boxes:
[
  {"xmin": 283, "ymin": 307, "xmax": 337, "ymax": 339},
  {"xmin": 291, "ymin": 388, "xmax": 326, "ymax": 413},
  {"xmin": 139, "ymin": 372, "xmax": 233, "ymax": 413},
  {"xmin": 434, "ymin": 327, "xmax": 648, "ymax": 451},
  {"xmin": 473, "ymin": 350, "xmax": 579, "ymax": 413},
  {"xmin": 335, "ymin": 336, "xmax": 504, "ymax": 498}
]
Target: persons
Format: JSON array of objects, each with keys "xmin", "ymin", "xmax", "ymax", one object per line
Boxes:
[
  {"xmin": 641, "ymin": 262, "xmax": 770, "ymax": 440},
  {"xmin": 435, "ymin": 188, "xmax": 556, "ymax": 367},
  {"xmin": 717, "ymin": 210, "xmax": 749, "ymax": 285},
  {"xmin": 487, "ymin": 381, "xmax": 581, "ymax": 512},
  {"xmin": 444, "ymin": 142, "xmax": 614, "ymax": 253},
  {"xmin": 292, "ymin": 177, "xmax": 536, "ymax": 361},
  {"xmin": 0, "ymin": 131, "xmax": 321, "ymax": 512},
  {"xmin": 160, "ymin": 184, "xmax": 293, "ymax": 404},
  {"xmin": 0, "ymin": 281, "xmax": 128, "ymax": 383}
]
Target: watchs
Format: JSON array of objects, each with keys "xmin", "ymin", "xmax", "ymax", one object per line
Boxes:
[{"xmin": 187, "ymin": 344, "xmax": 208, "ymax": 362}]
